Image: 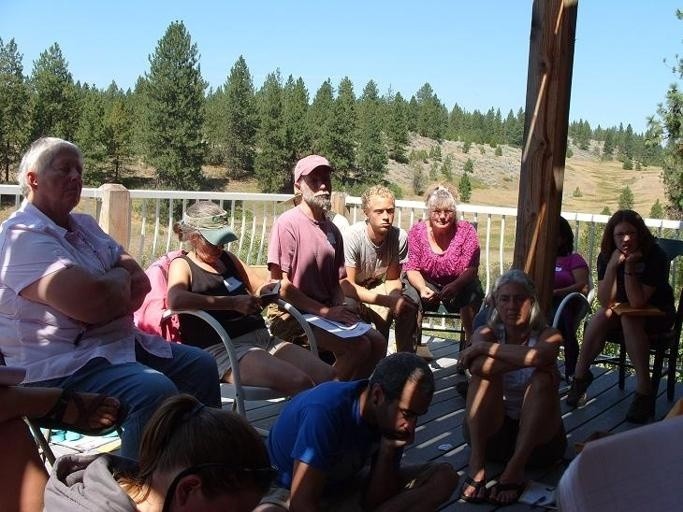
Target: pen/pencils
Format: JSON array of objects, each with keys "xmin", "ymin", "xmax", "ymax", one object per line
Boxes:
[{"xmin": 244, "ymin": 287, "xmax": 265, "ymax": 311}]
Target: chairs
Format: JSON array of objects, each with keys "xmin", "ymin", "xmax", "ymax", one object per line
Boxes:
[
  {"xmin": 589, "ymin": 240, "xmax": 681, "ymax": 404},
  {"xmin": 138, "ymin": 250, "xmax": 319, "ymax": 432}
]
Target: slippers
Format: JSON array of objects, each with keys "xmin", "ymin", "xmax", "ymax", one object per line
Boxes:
[
  {"xmin": 29, "ymin": 387, "xmax": 129, "ymax": 436},
  {"xmin": 485, "ymin": 481, "xmax": 524, "ymax": 506},
  {"xmin": 459, "ymin": 474, "xmax": 486, "ymax": 503}
]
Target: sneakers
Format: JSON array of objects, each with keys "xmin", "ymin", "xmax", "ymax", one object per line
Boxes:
[
  {"xmin": 565, "ymin": 371, "xmax": 593, "ymax": 406},
  {"xmin": 626, "ymin": 392, "xmax": 646, "ymax": 422}
]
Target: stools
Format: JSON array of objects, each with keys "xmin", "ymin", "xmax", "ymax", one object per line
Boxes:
[
  {"xmin": 21, "ymin": 411, "xmax": 124, "ymax": 469},
  {"xmin": 406, "ymin": 284, "xmax": 484, "ymax": 370}
]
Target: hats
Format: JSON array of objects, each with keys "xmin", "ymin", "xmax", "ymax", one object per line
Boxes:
[
  {"xmin": 295, "ymin": 155, "xmax": 332, "ymax": 184},
  {"xmin": 182, "ymin": 205, "xmax": 238, "ymax": 245}
]
